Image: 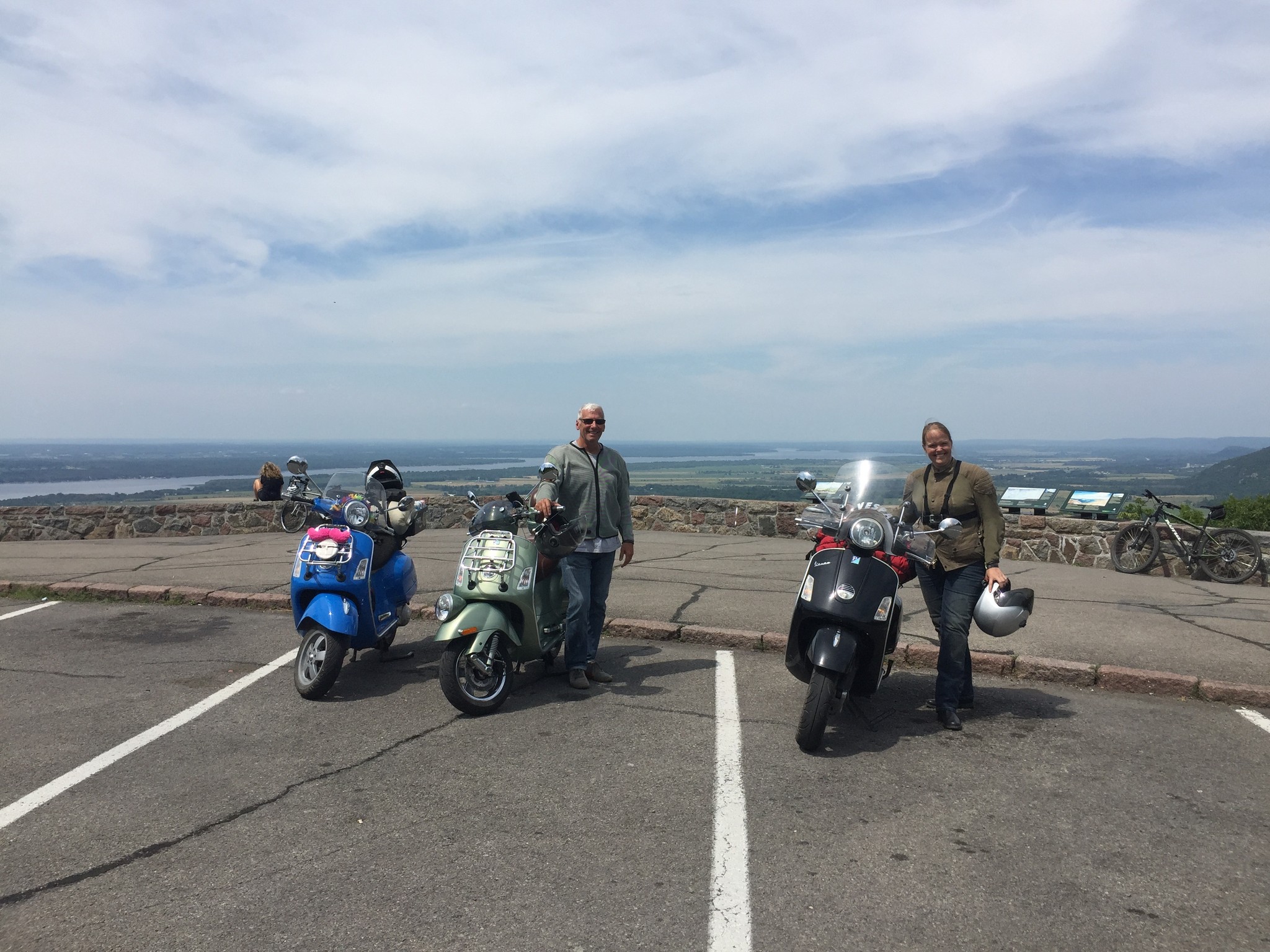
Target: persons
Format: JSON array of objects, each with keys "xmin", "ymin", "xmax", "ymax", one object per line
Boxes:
[
  {"xmin": 892, "ymin": 422, "xmax": 1008, "ymax": 729},
  {"xmin": 254, "ymin": 462, "xmax": 284, "ymax": 501},
  {"xmin": 535, "ymin": 403, "xmax": 634, "ymax": 690}
]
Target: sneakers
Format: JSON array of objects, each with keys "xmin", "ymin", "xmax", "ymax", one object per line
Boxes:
[
  {"xmin": 570, "ymin": 669, "xmax": 589, "ymax": 689},
  {"xmin": 585, "ymin": 661, "xmax": 612, "ymax": 682}
]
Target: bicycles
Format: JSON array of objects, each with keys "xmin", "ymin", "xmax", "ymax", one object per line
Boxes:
[
  {"xmin": 281, "ymin": 475, "xmax": 309, "ymax": 533},
  {"xmin": 1110, "ymin": 488, "xmax": 1261, "ymax": 583}
]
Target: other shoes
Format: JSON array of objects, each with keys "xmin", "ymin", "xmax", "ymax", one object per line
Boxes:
[{"xmin": 253, "ymin": 497, "xmax": 259, "ymax": 501}]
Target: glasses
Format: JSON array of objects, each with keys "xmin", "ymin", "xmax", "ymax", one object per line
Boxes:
[{"xmin": 578, "ymin": 418, "xmax": 605, "ymax": 425}]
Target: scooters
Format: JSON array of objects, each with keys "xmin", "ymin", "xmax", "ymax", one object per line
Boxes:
[
  {"xmin": 433, "ymin": 462, "xmax": 590, "ymax": 718},
  {"xmin": 794, "ymin": 459, "xmax": 963, "ymax": 752},
  {"xmin": 282, "ymin": 455, "xmax": 428, "ymax": 700}
]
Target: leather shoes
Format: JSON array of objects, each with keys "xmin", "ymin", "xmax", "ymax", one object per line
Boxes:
[
  {"xmin": 925, "ymin": 698, "xmax": 974, "ymax": 709},
  {"xmin": 938, "ymin": 710, "xmax": 962, "ymax": 729}
]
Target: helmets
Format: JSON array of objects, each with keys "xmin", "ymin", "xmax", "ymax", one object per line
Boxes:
[
  {"xmin": 534, "ymin": 512, "xmax": 587, "ymax": 560},
  {"xmin": 364, "ymin": 460, "xmax": 403, "ymax": 491},
  {"xmin": 972, "ymin": 576, "xmax": 1034, "ymax": 637}
]
